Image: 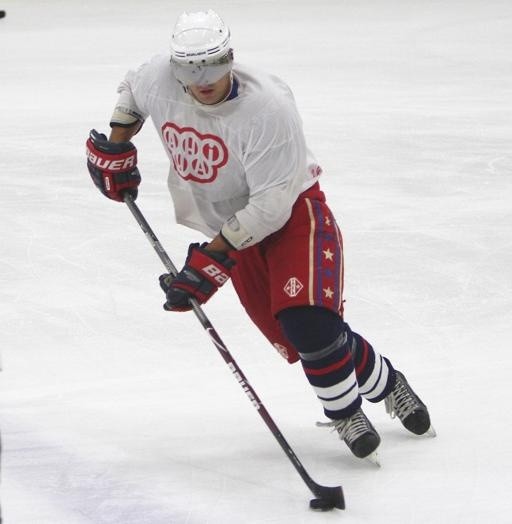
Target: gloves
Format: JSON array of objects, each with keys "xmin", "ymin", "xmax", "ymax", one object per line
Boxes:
[
  {"xmin": 86, "ymin": 129, "xmax": 141, "ymax": 202},
  {"xmin": 159, "ymin": 241, "xmax": 235, "ymax": 313}
]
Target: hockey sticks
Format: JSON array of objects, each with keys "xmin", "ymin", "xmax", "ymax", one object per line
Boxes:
[{"xmin": 123, "ymin": 191, "xmax": 345, "ymax": 510}]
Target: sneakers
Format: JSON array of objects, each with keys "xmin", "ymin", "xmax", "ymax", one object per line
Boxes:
[
  {"xmin": 386, "ymin": 371, "xmax": 430, "ymax": 434},
  {"xmin": 334, "ymin": 406, "xmax": 380, "ymax": 458}
]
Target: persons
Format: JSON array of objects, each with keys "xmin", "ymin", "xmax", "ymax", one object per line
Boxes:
[{"xmin": 86, "ymin": 6, "xmax": 430, "ymax": 460}]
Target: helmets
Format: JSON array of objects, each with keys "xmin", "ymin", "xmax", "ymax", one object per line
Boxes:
[{"xmin": 170, "ymin": 10, "xmax": 233, "ymax": 87}]
